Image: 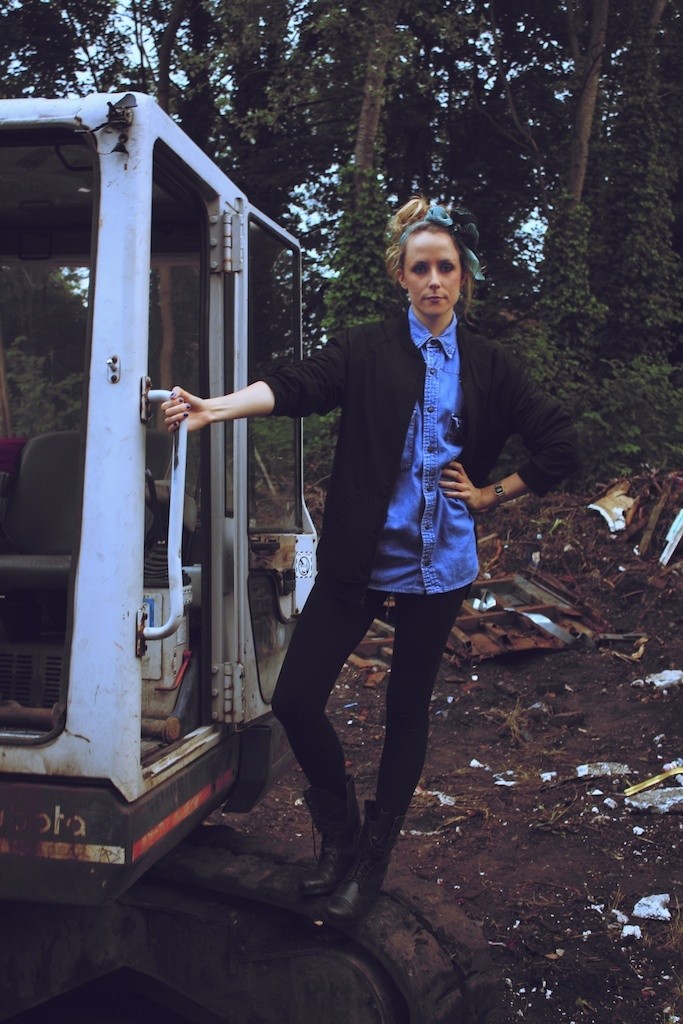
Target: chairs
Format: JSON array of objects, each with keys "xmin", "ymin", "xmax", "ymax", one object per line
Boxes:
[{"xmin": 9, "ymin": 432, "xmax": 174, "ymax": 558}]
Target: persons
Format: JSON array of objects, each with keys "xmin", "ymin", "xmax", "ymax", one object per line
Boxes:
[{"xmin": 162, "ymin": 195, "xmax": 590, "ymax": 926}]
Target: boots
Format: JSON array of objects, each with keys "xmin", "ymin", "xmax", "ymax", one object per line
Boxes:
[
  {"xmin": 298, "ymin": 773, "xmax": 360, "ymax": 896},
  {"xmin": 326, "ymin": 797, "xmax": 405, "ymax": 921}
]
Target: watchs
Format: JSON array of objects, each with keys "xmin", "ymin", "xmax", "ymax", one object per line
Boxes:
[{"xmin": 494, "ymin": 480, "xmax": 507, "ymax": 501}]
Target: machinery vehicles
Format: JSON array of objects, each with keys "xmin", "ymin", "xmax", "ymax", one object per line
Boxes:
[{"xmin": 0, "ymin": 89, "xmax": 500, "ymax": 1024}]
